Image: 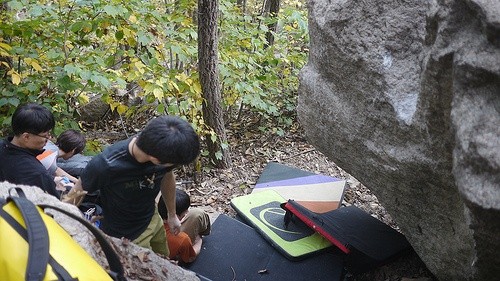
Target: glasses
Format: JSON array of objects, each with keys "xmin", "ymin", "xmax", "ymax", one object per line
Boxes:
[{"xmin": 24, "ymin": 131, "xmax": 51, "ymax": 143}]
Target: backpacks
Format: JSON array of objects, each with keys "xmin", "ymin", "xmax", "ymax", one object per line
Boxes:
[{"xmin": 0, "ymin": 186, "xmax": 126, "ymax": 281}]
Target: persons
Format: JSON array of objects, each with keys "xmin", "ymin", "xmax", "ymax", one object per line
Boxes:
[
  {"xmin": 61, "ymin": 115, "xmax": 200, "ymax": 259},
  {"xmin": 34, "ymin": 128, "xmax": 86, "ymax": 184},
  {"xmin": 0, "ymin": 102, "xmax": 67, "ymax": 202},
  {"xmin": 157, "ymin": 188, "xmax": 210, "ymax": 264}
]
